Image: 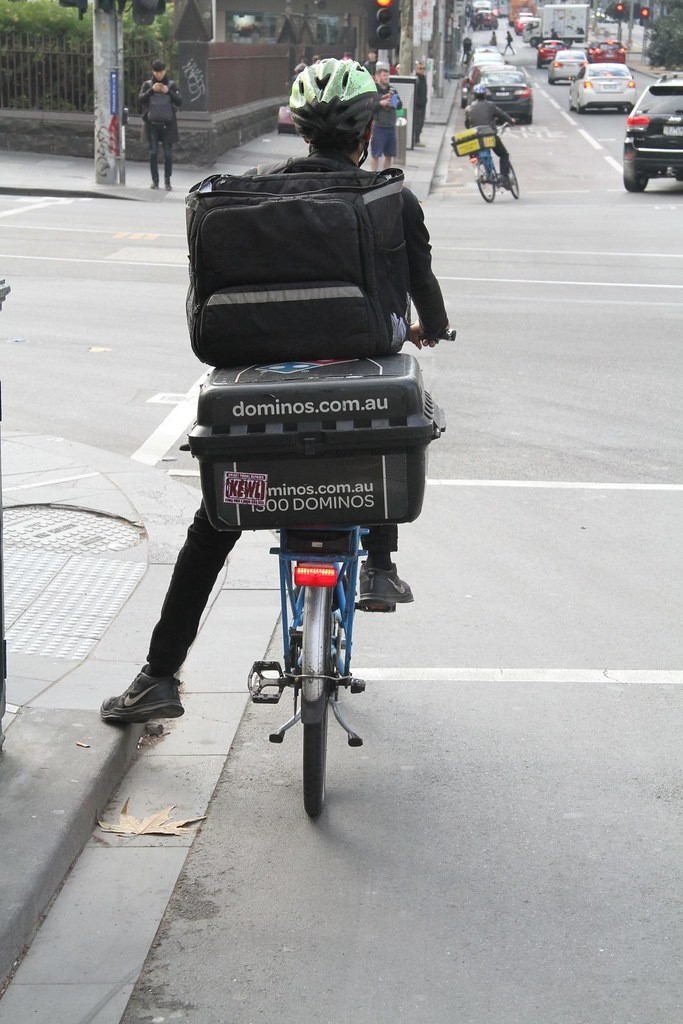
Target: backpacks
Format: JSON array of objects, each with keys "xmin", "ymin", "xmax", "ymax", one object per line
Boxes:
[
  {"xmin": 184, "ymin": 161, "xmax": 411, "ymax": 369},
  {"xmin": 146, "ymin": 79, "xmax": 174, "ymax": 125}
]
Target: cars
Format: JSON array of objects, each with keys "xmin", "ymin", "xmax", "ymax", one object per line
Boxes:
[
  {"xmin": 472, "ymin": 10, "xmax": 499, "ymax": 30},
  {"xmin": 545, "ymin": 50, "xmax": 590, "ymax": 85},
  {"xmin": 584, "ymin": 40, "xmax": 628, "ymax": 65},
  {"xmin": 459, "ymin": 46, "xmax": 521, "ymax": 109},
  {"xmin": 467, "ymin": 69, "xmax": 534, "ymax": 125},
  {"xmin": 535, "ymin": 39, "xmax": 570, "ymax": 68},
  {"xmin": 590, "ymin": 7, "xmax": 618, "ymax": 24},
  {"xmin": 568, "ymin": 62, "xmax": 638, "ymax": 115},
  {"xmin": 513, "ymin": 12, "xmax": 534, "ymax": 35}
]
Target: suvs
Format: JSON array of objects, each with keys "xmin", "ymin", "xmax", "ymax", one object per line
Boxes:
[{"xmin": 617, "ymin": 72, "xmax": 683, "ymax": 192}]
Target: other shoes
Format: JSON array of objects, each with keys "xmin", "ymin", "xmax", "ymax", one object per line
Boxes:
[
  {"xmin": 165, "ymin": 182, "xmax": 172, "ymax": 191},
  {"xmin": 417, "ymin": 142, "xmax": 426, "ymax": 147},
  {"xmin": 150, "ymin": 183, "xmax": 159, "ymax": 188},
  {"xmin": 500, "ymin": 175, "xmax": 513, "ymax": 191}
]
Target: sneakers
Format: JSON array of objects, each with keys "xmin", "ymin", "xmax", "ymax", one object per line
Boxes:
[
  {"xmin": 359, "ymin": 560, "xmax": 414, "ymax": 606},
  {"xmin": 100, "ymin": 664, "xmax": 184, "ymax": 724}
]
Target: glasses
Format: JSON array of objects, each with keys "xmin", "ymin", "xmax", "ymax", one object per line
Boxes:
[{"xmin": 417, "ymin": 67, "xmax": 426, "ymax": 70}]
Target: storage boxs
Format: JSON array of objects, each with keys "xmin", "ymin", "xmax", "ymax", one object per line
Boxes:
[
  {"xmin": 451, "ymin": 125, "xmax": 496, "ymax": 155},
  {"xmin": 184, "ymin": 351, "xmax": 447, "ymax": 530}
]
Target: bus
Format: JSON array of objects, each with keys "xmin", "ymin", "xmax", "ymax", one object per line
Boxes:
[{"xmin": 466, "ymin": 0, "xmax": 537, "ymax": 27}]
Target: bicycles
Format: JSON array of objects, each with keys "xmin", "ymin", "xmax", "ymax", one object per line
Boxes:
[
  {"xmin": 451, "ymin": 122, "xmax": 520, "ymax": 203},
  {"xmin": 179, "ymin": 327, "xmax": 460, "ymax": 822}
]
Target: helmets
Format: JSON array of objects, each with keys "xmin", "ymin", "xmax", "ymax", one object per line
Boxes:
[
  {"xmin": 288, "ymin": 58, "xmax": 378, "ymax": 144},
  {"xmin": 472, "ymin": 83, "xmax": 487, "ymax": 97}
]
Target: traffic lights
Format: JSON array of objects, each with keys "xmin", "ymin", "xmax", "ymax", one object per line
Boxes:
[
  {"xmin": 58, "ymin": 0, "xmax": 88, "ymax": 21},
  {"xmin": 614, "ymin": 3, "xmax": 625, "ymax": 19},
  {"xmin": 367, "ymin": 0, "xmax": 401, "ymax": 50},
  {"xmin": 639, "ymin": 7, "xmax": 651, "ymax": 26},
  {"xmin": 133, "ymin": 0, "xmax": 166, "ymax": 26}
]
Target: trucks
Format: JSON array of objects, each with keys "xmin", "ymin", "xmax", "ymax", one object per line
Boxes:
[{"xmin": 521, "ymin": 3, "xmax": 591, "ymax": 49}]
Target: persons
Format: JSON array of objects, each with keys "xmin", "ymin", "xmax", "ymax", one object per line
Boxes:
[
  {"xmin": 371, "ymin": 69, "xmax": 402, "ymax": 172},
  {"xmin": 550, "ymin": 29, "xmax": 557, "ymax": 39},
  {"xmin": 99, "ymin": 57, "xmax": 447, "ymax": 724},
  {"xmin": 463, "ymin": 37, "xmax": 472, "ymax": 61},
  {"xmin": 504, "ymin": 30, "xmax": 514, "ymax": 54},
  {"xmin": 464, "ymin": 82, "xmax": 515, "ymax": 190},
  {"xmin": 138, "ymin": 61, "xmax": 183, "ymax": 191},
  {"xmin": 490, "ymin": 31, "xmax": 496, "ymax": 46},
  {"xmin": 290, "ymin": 51, "xmax": 400, "ymax": 82},
  {"xmin": 411, "ymin": 61, "xmax": 427, "ymax": 147}
]
